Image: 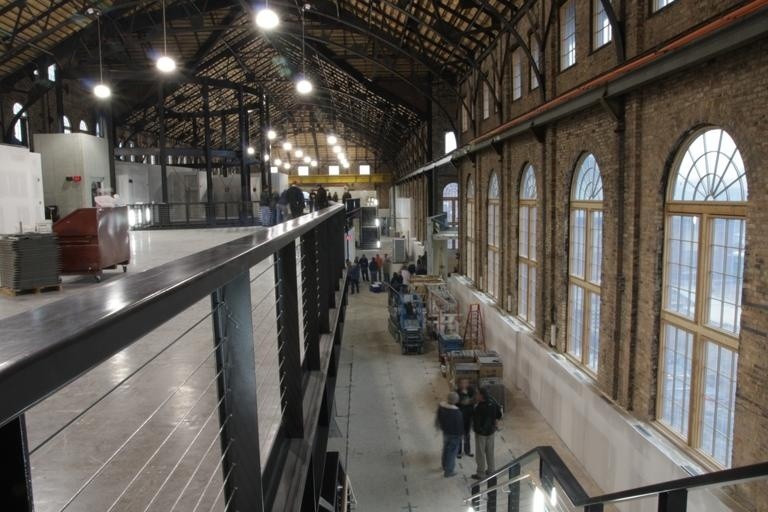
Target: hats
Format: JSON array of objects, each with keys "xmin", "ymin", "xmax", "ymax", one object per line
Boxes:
[{"xmin": 446, "ymin": 392, "xmax": 459, "ymax": 404}]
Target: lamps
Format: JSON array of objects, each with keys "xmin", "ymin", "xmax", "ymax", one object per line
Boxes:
[
  {"xmin": 255, "ymin": 0, "xmax": 280, "ymax": 30},
  {"xmin": 155, "ymin": 0, "xmax": 177, "ymax": 73},
  {"xmin": 92, "ymin": 12, "xmax": 113, "ymax": 100},
  {"xmin": 297, "ymin": 5, "xmax": 313, "ymax": 95}
]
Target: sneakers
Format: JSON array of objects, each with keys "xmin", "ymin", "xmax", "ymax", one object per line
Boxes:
[{"xmin": 456, "ymin": 451, "xmax": 475, "ymax": 458}]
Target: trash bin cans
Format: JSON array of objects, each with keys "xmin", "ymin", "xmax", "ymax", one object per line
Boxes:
[{"xmin": 438, "ymin": 334, "xmax": 464, "ymax": 364}]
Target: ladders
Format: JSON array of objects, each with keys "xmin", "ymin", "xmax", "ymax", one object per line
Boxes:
[{"xmin": 463, "ymin": 303, "xmax": 486, "ymax": 351}]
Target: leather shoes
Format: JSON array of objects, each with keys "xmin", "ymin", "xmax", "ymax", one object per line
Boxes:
[
  {"xmin": 470, "ymin": 471, "xmax": 497, "ymax": 480},
  {"xmin": 442, "ymin": 464, "xmax": 459, "ymax": 477}
]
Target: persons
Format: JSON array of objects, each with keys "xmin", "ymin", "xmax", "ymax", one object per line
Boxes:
[
  {"xmin": 435, "ymin": 375, "xmax": 502, "ymax": 480},
  {"xmin": 251, "ymin": 180, "xmax": 351, "ymax": 227},
  {"xmin": 345, "ymin": 250, "xmax": 427, "ymax": 294}
]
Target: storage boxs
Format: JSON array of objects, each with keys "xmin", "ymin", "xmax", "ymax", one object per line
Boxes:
[{"xmin": 439, "ymin": 333, "xmax": 505, "ymax": 412}]
